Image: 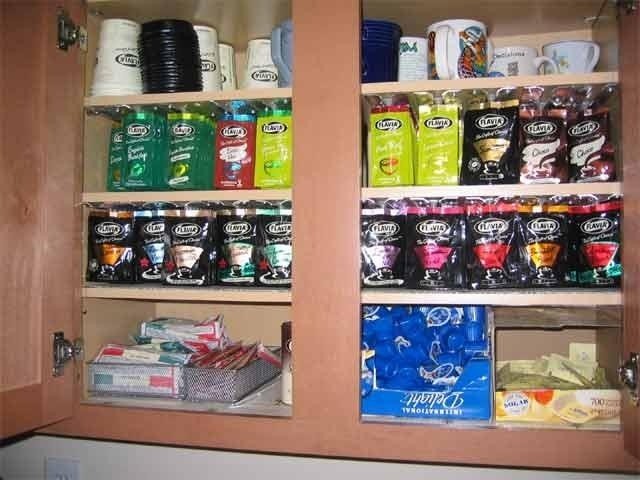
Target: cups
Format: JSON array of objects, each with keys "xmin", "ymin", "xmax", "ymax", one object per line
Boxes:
[
  {"xmin": 361, "ymin": 19, "xmax": 602, "ymax": 82},
  {"xmin": 89, "ymin": 18, "xmax": 293, "ymax": 96}
]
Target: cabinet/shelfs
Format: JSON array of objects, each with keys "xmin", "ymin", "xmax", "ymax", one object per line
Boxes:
[
  {"xmin": 0, "ymin": 1, "xmax": 324, "ymax": 456},
  {"xmin": 325, "ymin": 0, "xmax": 640, "ymax": 474}
]
